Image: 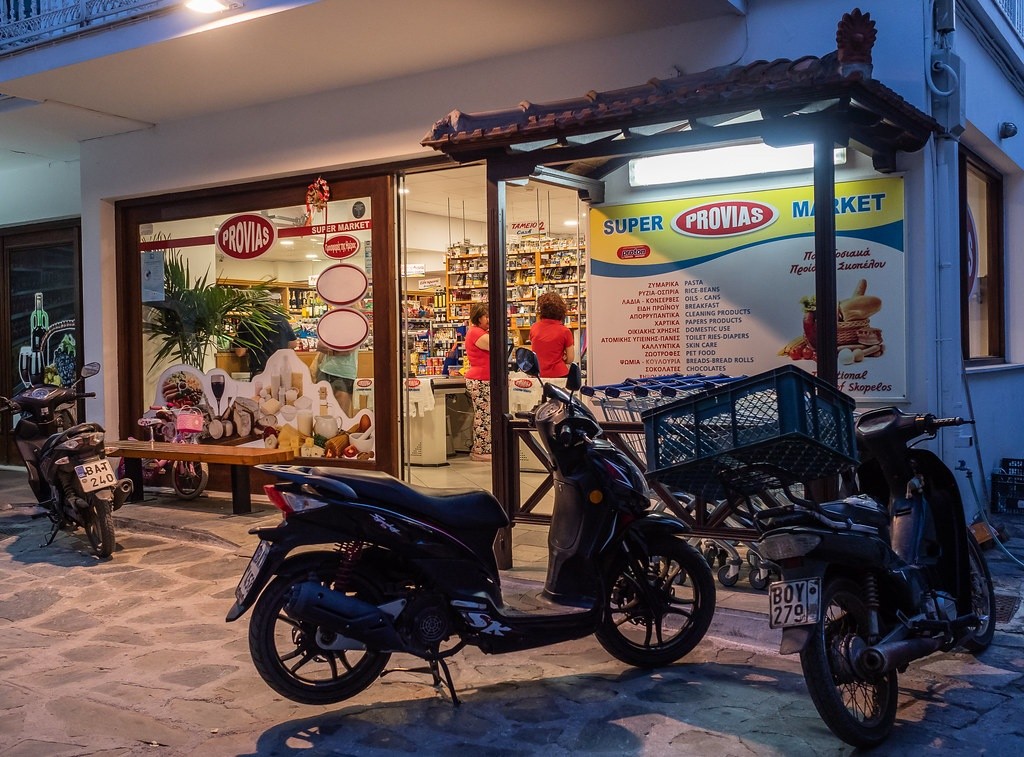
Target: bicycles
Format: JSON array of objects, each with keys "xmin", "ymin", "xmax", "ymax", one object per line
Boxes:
[{"xmin": 118, "ymin": 402, "xmax": 208, "ymax": 500}]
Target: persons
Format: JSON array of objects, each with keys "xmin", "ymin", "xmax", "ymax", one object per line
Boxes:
[
  {"xmin": 529, "ymin": 292, "xmax": 575, "ymax": 378},
  {"xmin": 465, "ymin": 302, "xmax": 492, "ymax": 462},
  {"xmin": 232, "ymin": 290, "xmax": 297, "ymax": 382},
  {"xmin": 315, "ymin": 305, "xmax": 359, "ymax": 418}
]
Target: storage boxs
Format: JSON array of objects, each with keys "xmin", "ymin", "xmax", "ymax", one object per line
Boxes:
[
  {"xmin": 639, "ymin": 364, "xmax": 860, "ymax": 499},
  {"xmin": 991, "ymin": 457, "xmax": 1024, "ymax": 516}
]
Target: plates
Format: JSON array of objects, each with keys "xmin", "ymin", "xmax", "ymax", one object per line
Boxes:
[
  {"xmin": 289, "ymin": 290, "xmax": 327, "ymax": 318},
  {"xmin": 209, "ymin": 420, "xmax": 223, "ymax": 439}
]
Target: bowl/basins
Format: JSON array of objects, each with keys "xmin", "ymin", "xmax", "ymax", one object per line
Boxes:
[
  {"xmin": 349, "ymin": 432, "xmax": 374, "ymax": 453},
  {"xmin": 294, "ymin": 395, "xmax": 313, "ymax": 410},
  {"xmin": 280, "ymin": 404, "xmax": 297, "ymax": 421}
]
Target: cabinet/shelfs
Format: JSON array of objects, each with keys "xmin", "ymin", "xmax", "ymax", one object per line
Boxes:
[{"xmin": 216, "ymin": 240, "xmax": 586, "ymax": 377}]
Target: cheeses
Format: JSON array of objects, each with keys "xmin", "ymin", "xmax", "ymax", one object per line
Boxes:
[{"xmin": 232, "ymin": 397, "xmax": 325, "ymax": 457}]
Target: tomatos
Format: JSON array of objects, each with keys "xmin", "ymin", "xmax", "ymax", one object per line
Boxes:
[{"xmin": 789, "ymin": 346, "xmax": 813, "ymax": 360}]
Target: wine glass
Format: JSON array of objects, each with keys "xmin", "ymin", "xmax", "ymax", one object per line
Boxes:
[{"xmin": 211, "ymin": 374, "xmax": 226, "ymax": 418}]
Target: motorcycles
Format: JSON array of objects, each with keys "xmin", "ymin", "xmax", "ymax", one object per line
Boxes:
[
  {"xmin": 720, "ymin": 406, "xmax": 996, "ymax": 750},
  {"xmin": 0, "ymin": 361, "xmax": 134, "ymax": 558}
]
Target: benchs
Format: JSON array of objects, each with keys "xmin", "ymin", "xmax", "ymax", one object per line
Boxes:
[{"xmin": 104, "ymin": 440, "xmax": 295, "ymax": 516}]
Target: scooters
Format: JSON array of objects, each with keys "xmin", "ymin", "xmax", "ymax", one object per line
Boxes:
[{"xmin": 224, "ymin": 348, "xmax": 716, "ymax": 707}]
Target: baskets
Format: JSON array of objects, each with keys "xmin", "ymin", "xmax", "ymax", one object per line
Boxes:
[
  {"xmin": 176, "ymin": 413, "xmax": 203, "ymax": 431},
  {"xmin": 803, "ymin": 319, "xmax": 870, "ymax": 352}
]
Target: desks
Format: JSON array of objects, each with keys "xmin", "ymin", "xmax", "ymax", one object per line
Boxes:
[{"xmin": 353, "ymin": 378, "xmax": 474, "ymax": 467}]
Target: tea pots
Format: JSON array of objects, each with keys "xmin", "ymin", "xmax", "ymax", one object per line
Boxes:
[{"xmin": 315, "ymin": 415, "xmax": 343, "ymax": 439}]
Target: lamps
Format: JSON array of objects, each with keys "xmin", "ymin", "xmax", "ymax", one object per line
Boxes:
[
  {"xmin": 183, "ymin": 0, "xmax": 243, "ymax": 15},
  {"xmin": 629, "ymin": 139, "xmax": 848, "ymax": 189}
]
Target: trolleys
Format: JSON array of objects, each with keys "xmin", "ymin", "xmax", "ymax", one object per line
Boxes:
[{"xmin": 584, "ymin": 372, "xmax": 804, "ymax": 589}]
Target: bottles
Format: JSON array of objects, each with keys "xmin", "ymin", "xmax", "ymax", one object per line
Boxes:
[
  {"xmin": 313, "ymin": 386, "xmax": 329, "ymax": 434},
  {"xmin": 433, "ymin": 328, "xmax": 453, "ymax": 356},
  {"xmin": 30, "ymin": 293, "xmax": 49, "ymax": 375},
  {"xmin": 434, "ymin": 286, "xmax": 446, "ymax": 308}
]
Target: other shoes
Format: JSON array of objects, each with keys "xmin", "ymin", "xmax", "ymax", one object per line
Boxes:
[{"xmin": 470, "ymin": 454, "xmax": 492, "ymax": 462}]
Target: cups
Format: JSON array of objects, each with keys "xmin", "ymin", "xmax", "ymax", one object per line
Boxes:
[
  {"xmin": 297, "ymin": 409, "xmax": 313, "ymax": 437},
  {"xmin": 286, "ymin": 390, "xmax": 297, "ymax": 406},
  {"xmin": 19, "ymin": 347, "xmax": 31, "ymax": 389}
]
